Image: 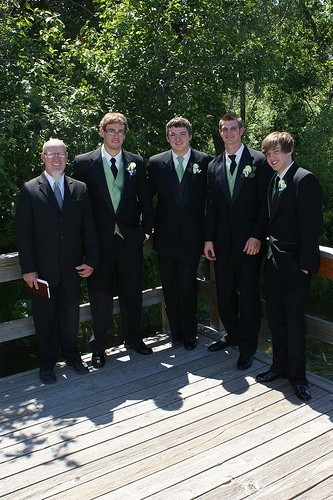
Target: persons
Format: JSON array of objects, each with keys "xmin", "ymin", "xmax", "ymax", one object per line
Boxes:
[
  {"xmin": 17, "ymin": 138, "xmax": 99, "ymax": 384},
  {"xmin": 255, "ymin": 131, "xmax": 322, "ymax": 402},
  {"xmin": 70, "ymin": 112, "xmax": 153, "ymax": 369},
  {"xmin": 146, "ymin": 116, "xmax": 215, "ymax": 350},
  {"xmin": 204, "ymin": 110, "xmax": 266, "ymax": 370}
]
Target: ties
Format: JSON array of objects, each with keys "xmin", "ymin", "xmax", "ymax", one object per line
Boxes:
[
  {"xmin": 110, "ymin": 158, "xmax": 118, "ymax": 181},
  {"xmin": 54, "ymin": 180, "xmax": 62, "ymax": 211},
  {"xmin": 176, "ymin": 156, "xmax": 185, "ymax": 183},
  {"xmin": 228, "ymin": 155, "xmax": 237, "ymax": 176},
  {"xmin": 273, "ymin": 176, "xmax": 280, "ymax": 209}
]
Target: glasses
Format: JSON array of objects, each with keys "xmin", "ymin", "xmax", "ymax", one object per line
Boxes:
[
  {"xmin": 44, "ymin": 152, "xmax": 66, "ymax": 158},
  {"xmin": 104, "ymin": 129, "xmax": 125, "ymax": 134}
]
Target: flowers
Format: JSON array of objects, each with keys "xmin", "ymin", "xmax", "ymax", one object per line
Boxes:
[
  {"xmin": 242, "ymin": 165, "xmax": 252, "ymax": 178},
  {"xmin": 126, "ymin": 162, "xmax": 137, "ymax": 176},
  {"xmin": 192, "ymin": 164, "xmax": 202, "ymax": 176},
  {"xmin": 277, "ymin": 179, "xmax": 287, "ymax": 192}
]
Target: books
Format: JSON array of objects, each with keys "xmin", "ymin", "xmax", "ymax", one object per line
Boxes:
[{"xmin": 25, "ymin": 278, "xmax": 50, "ymax": 298}]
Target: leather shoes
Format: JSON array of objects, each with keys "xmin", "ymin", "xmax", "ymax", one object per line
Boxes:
[
  {"xmin": 185, "ymin": 340, "xmax": 197, "ymax": 351},
  {"xmin": 256, "ymin": 370, "xmax": 288, "ymax": 382},
  {"xmin": 40, "ymin": 367, "xmax": 56, "ymax": 383},
  {"xmin": 66, "ymin": 358, "xmax": 88, "ymax": 374},
  {"xmin": 125, "ymin": 340, "xmax": 150, "ymax": 355},
  {"xmin": 208, "ymin": 339, "xmax": 238, "ymax": 351},
  {"xmin": 237, "ymin": 352, "xmax": 253, "ymax": 370},
  {"xmin": 172, "ymin": 338, "xmax": 183, "ymax": 347},
  {"xmin": 92, "ymin": 352, "xmax": 105, "ymax": 368},
  {"xmin": 292, "ymin": 384, "xmax": 311, "ymax": 400}
]
